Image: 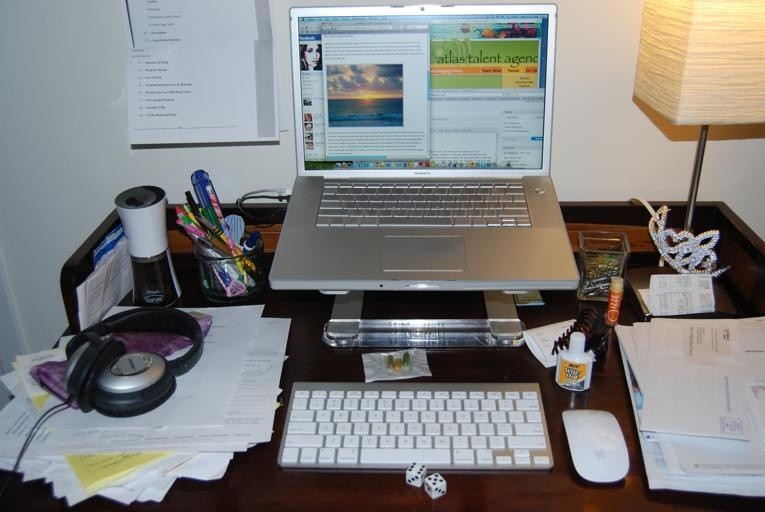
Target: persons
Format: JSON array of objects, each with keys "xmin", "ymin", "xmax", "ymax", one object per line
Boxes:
[{"xmin": 300, "ymin": 44, "xmax": 322, "ymax": 71}]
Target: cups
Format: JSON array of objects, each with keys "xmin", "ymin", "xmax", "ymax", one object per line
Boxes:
[{"xmin": 574, "ymin": 230, "xmax": 631, "ymax": 301}]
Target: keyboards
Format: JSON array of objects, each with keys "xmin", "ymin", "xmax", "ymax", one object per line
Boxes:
[{"xmin": 277, "ymin": 382, "xmax": 554, "ymax": 471}]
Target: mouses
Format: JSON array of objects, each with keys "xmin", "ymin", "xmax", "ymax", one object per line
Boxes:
[{"xmin": 562, "ymin": 409, "xmax": 630, "ymax": 484}]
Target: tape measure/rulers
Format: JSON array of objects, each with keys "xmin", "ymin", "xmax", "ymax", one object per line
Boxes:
[{"xmin": 191, "ymin": 170, "xmax": 221, "ymax": 209}]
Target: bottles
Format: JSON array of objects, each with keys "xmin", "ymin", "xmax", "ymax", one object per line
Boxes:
[{"xmin": 553, "ymin": 330, "xmax": 595, "ymax": 394}]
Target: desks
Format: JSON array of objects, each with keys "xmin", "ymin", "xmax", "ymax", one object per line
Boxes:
[{"xmin": 6, "ymin": 200, "xmax": 763, "ymax": 504}]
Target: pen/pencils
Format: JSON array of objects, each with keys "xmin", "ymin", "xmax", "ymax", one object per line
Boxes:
[{"xmin": 176, "ymin": 186, "xmax": 260, "ymax": 297}]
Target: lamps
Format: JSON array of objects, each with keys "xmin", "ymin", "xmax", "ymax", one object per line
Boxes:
[{"xmin": 631, "ymin": 0, "xmax": 764, "ymax": 266}]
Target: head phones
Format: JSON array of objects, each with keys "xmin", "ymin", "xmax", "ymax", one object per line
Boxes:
[{"xmin": 62, "ymin": 306, "xmax": 204, "ymax": 418}]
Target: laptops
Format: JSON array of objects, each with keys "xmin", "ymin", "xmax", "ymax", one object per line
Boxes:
[{"xmin": 269, "ymin": 4, "xmax": 581, "ymax": 296}]
